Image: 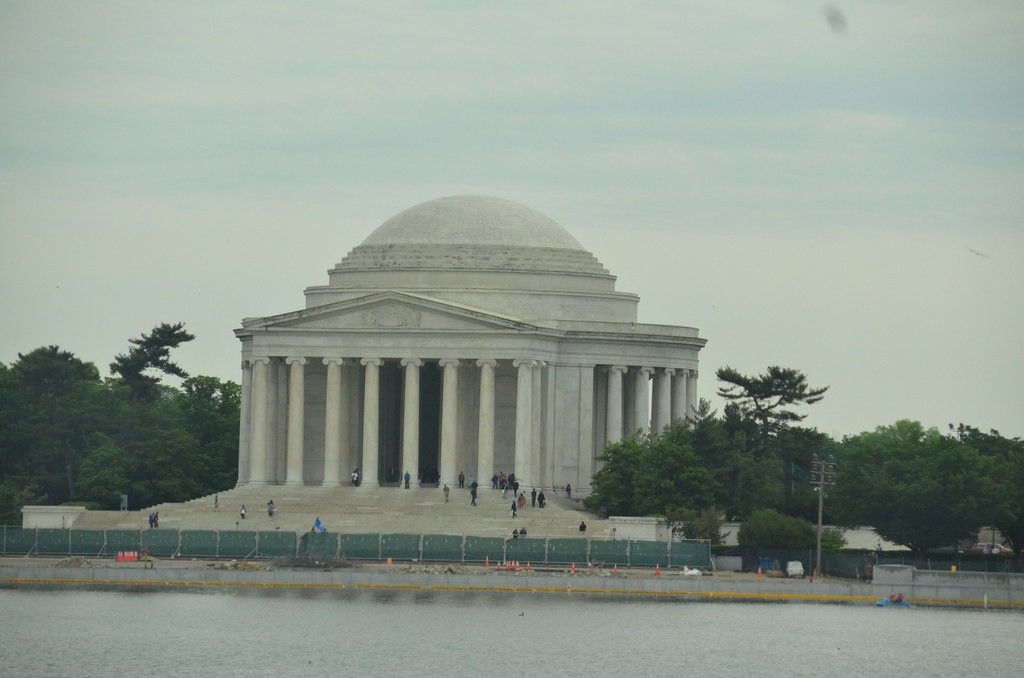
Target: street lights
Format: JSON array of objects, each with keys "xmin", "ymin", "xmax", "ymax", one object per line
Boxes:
[{"xmin": 809, "ymin": 453, "xmax": 837, "ymax": 578}]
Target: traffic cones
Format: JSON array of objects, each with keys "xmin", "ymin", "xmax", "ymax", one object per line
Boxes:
[
  {"xmin": 117, "ymin": 550, "xmax": 138, "ymax": 562},
  {"xmin": 654, "ymin": 564, "xmax": 660, "ymax": 575},
  {"xmin": 757, "ymin": 568, "xmax": 763, "ymax": 577},
  {"xmin": 484, "ymin": 556, "xmax": 489, "ymax": 567},
  {"xmin": 614, "ymin": 564, "xmax": 618, "ymax": 574},
  {"xmin": 496, "ymin": 560, "xmax": 531, "ymax": 572},
  {"xmin": 572, "ymin": 563, "xmax": 575, "ymax": 573}
]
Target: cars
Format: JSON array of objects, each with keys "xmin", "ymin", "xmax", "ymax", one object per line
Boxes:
[{"xmin": 973, "ymin": 542, "xmax": 1011, "ymax": 553}]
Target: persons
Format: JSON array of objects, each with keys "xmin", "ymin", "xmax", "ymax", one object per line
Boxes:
[
  {"xmin": 214, "ymin": 495, "xmax": 220, "ymax": 512},
  {"xmin": 468, "ymin": 475, "xmax": 478, "ymax": 506},
  {"xmin": 315, "ymin": 517, "xmax": 321, "ymax": 528},
  {"xmin": 992, "ymin": 545, "xmax": 999, "ymax": 553},
  {"xmin": 240, "ymin": 505, "xmax": 246, "ymax": 519},
  {"xmin": 580, "ymin": 521, "xmax": 586, "ymax": 531},
  {"xmin": 492, "ymin": 472, "xmax": 527, "ymax": 518},
  {"xmin": 404, "ymin": 471, "xmax": 410, "ymax": 489},
  {"xmin": 889, "ymin": 593, "xmax": 903, "ymax": 603},
  {"xmin": 531, "ymin": 488, "xmax": 545, "ymax": 509},
  {"xmin": 435, "ymin": 472, "xmax": 440, "ymax": 487},
  {"xmin": 267, "ymin": 500, "xmax": 273, "ymax": 517},
  {"xmin": 513, "ymin": 527, "xmax": 527, "ymax": 538},
  {"xmin": 149, "ymin": 512, "xmax": 159, "ymax": 527},
  {"xmin": 444, "ymin": 484, "xmax": 449, "ymax": 502},
  {"xmin": 458, "ymin": 471, "xmax": 465, "ymax": 488},
  {"xmin": 875, "ymin": 544, "xmax": 882, "ymax": 551},
  {"xmin": 566, "ymin": 484, "xmax": 571, "ymax": 498},
  {"xmin": 351, "ymin": 469, "xmax": 359, "ymax": 486}
]
